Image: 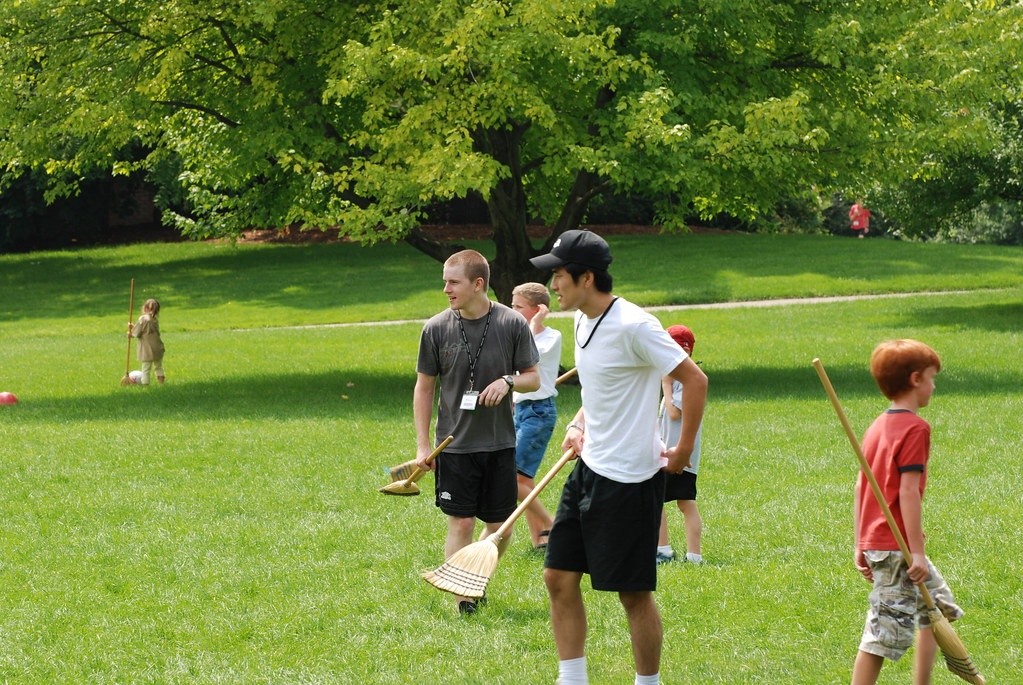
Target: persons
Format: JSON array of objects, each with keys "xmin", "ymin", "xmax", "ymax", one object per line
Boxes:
[
  {"xmin": 852, "ymin": 339, "xmax": 966, "ymax": 685},
  {"xmin": 849, "ymin": 200, "xmax": 870, "ymax": 238},
  {"xmin": 413, "ymin": 249, "xmax": 539, "ymax": 614},
  {"xmin": 528, "ymin": 227, "xmax": 707, "ymax": 685},
  {"xmin": 511, "ymin": 283, "xmax": 555, "ymax": 551},
  {"xmin": 657, "ymin": 324, "xmax": 702, "ymax": 565},
  {"xmin": 128, "ymin": 299, "xmax": 165, "ymax": 385}
]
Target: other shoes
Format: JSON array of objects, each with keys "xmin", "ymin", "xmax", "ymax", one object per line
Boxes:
[{"xmin": 459, "ymin": 597, "xmax": 488, "ymax": 616}]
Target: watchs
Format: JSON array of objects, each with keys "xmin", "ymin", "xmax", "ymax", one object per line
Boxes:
[{"xmin": 502, "ymin": 375, "xmax": 514, "ymax": 391}]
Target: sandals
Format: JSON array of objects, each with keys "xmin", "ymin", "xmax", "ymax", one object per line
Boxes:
[{"xmin": 536, "ymin": 530, "xmax": 550, "ymax": 548}]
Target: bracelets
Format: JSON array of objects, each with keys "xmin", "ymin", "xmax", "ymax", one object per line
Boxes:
[{"xmin": 566, "ymin": 421, "xmax": 584, "ymax": 431}]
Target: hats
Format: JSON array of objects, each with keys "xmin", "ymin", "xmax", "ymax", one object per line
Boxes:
[
  {"xmin": 529, "ymin": 228, "xmax": 613, "ymax": 273},
  {"xmin": 666, "ymin": 324, "xmax": 695, "ymax": 352}
]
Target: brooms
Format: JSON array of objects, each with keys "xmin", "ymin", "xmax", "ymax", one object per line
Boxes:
[
  {"xmin": 378, "ymin": 435, "xmax": 454, "ymax": 496},
  {"xmin": 118, "ymin": 278, "xmax": 135, "ymax": 385},
  {"xmin": 812, "ymin": 357, "xmax": 987, "ymax": 685},
  {"xmin": 392, "ymin": 366, "xmax": 578, "ymax": 486},
  {"xmin": 418, "ymin": 446, "xmax": 575, "ymax": 600}
]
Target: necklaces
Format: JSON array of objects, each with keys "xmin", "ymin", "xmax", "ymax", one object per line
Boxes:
[{"xmin": 576, "ymin": 297, "xmax": 619, "ymax": 348}]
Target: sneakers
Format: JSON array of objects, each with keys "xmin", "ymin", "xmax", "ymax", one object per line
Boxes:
[
  {"xmin": 680, "ymin": 556, "xmax": 704, "ymax": 564},
  {"xmin": 656, "ymin": 550, "xmax": 676, "ymax": 564}
]
